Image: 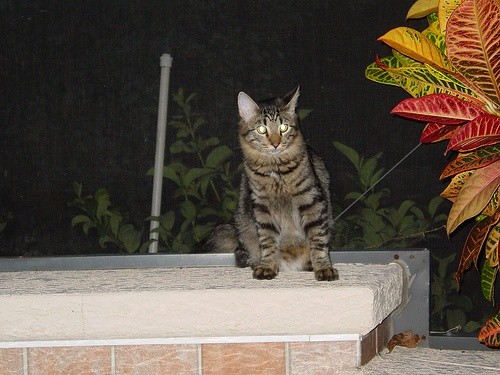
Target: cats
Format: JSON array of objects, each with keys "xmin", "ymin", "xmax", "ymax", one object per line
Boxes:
[{"xmin": 189, "ymin": 83, "xmax": 339, "ymax": 282}]
